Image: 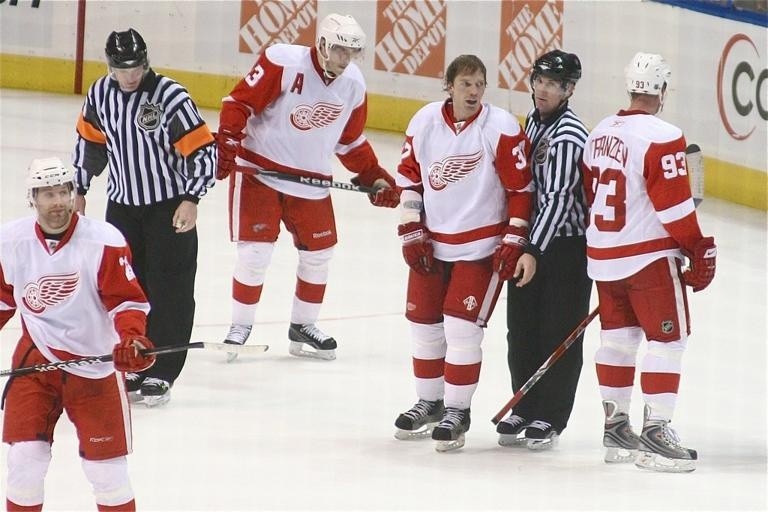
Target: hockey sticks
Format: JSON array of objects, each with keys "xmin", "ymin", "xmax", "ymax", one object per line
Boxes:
[
  {"xmin": 1, "ymin": 342, "xmax": 268, "ymax": 377},
  {"xmin": 491, "ymin": 144, "xmax": 704, "ymax": 425}
]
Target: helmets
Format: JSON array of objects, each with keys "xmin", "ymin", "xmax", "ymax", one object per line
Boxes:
[
  {"xmin": 624, "ymin": 51, "xmax": 673, "ymax": 95},
  {"xmin": 314, "ymin": 13, "xmax": 366, "ymax": 50},
  {"xmin": 104, "ymin": 28, "xmax": 149, "ymax": 71},
  {"xmin": 532, "ymin": 50, "xmax": 582, "ymax": 85},
  {"xmin": 25, "ymin": 156, "xmax": 80, "ymax": 207}
]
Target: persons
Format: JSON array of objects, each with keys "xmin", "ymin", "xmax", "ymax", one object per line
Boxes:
[
  {"xmin": 215, "ymin": 13, "xmax": 401, "ymax": 351},
  {"xmin": 395, "ymin": 54, "xmax": 536, "ymax": 440},
  {"xmin": 73, "ymin": 29, "xmax": 219, "ymax": 397},
  {"xmin": 496, "ymin": 50, "xmax": 593, "ymax": 440},
  {"xmin": 580, "ymin": 53, "xmax": 715, "ymax": 459},
  {"xmin": 0, "ymin": 157, "xmax": 156, "ymax": 512}
]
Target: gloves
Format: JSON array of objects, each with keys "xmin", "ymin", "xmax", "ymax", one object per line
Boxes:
[
  {"xmin": 493, "ymin": 224, "xmax": 531, "ymax": 282},
  {"xmin": 678, "ymin": 236, "xmax": 717, "ymax": 293},
  {"xmin": 213, "ymin": 126, "xmax": 247, "ymax": 181},
  {"xmin": 113, "ymin": 336, "xmax": 157, "ymax": 374},
  {"xmin": 397, "ymin": 222, "xmax": 434, "ymax": 275},
  {"xmin": 350, "ymin": 164, "xmax": 401, "ymax": 209}
]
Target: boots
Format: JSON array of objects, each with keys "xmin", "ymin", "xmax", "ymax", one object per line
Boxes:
[
  {"xmin": 601, "ymin": 399, "xmax": 639, "ymax": 450},
  {"xmin": 638, "ymin": 402, "xmax": 699, "ymax": 460}
]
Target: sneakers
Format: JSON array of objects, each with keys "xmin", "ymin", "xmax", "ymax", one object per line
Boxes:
[
  {"xmin": 495, "ymin": 415, "xmax": 527, "ymax": 435},
  {"xmin": 223, "ymin": 321, "xmax": 253, "ymax": 345},
  {"xmin": 124, "ymin": 374, "xmax": 142, "ymax": 392},
  {"xmin": 433, "ymin": 406, "xmax": 471, "ymax": 440},
  {"xmin": 394, "ymin": 397, "xmax": 445, "ymax": 431},
  {"xmin": 141, "ymin": 377, "xmax": 174, "ymax": 395},
  {"xmin": 288, "ymin": 322, "xmax": 338, "ymax": 350},
  {"xmin": 524, "ymin": 420, "xmax": 558, "ymax": 439}
]
